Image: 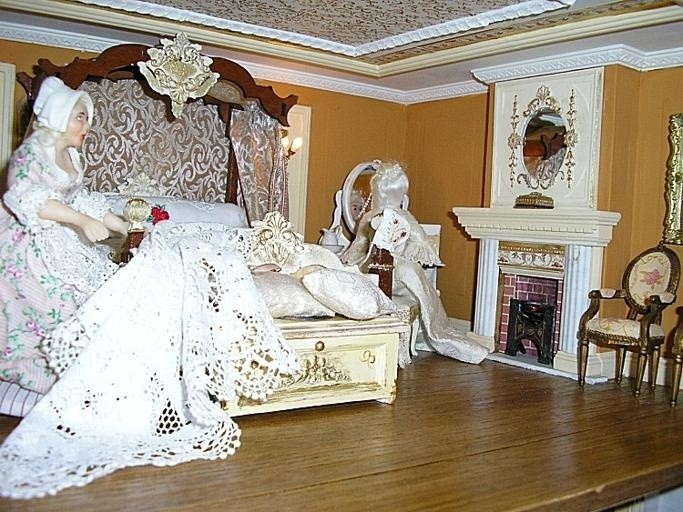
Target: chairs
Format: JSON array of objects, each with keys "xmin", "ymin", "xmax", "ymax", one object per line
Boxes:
[
  {"xmin": 663, "ymin": 304, "xmax": 682, "ymax": 411},
  {"xmin": 577, "ymin": 244, "xmax": 679, "ymax": 414}
]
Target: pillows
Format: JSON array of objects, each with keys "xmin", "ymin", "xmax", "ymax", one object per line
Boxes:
[
  {"xmin": 304, "ymin": 262, "xmax": 399, "ymax": 321},
  {"xmin": 254, "ymin": 268, "xmax": 335, "ymax": 323}
]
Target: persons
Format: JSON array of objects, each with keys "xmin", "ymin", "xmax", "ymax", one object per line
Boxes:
[
  {"xmin": 341, "ymin": 161, "xmax": 442, "ymax": 305},
  {"xmin": 350, "ymin": 192, "xmax": 365, "ymax": 221},
  {"xmin": 0, "ymin": 76, "xmax": 128, "ymax": 419}
]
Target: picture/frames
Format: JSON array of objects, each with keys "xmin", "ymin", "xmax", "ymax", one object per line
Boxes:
[{"xmin": 664, "ymin": 110, "xmax": 683, "ymax": 245}]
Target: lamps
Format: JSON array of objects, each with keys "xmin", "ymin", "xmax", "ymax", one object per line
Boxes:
[
  {"xmin": 279, "ymin": 133, "xmax": 303, "ymax": 161},
  {"xmin": 123, "ymin": 198, "xmax": 151, "ymax": 248}
]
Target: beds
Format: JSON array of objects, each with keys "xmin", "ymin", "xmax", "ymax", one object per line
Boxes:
[{"xmin": 2, "ymin": 32, "xmax": 405, "ymax": 512}]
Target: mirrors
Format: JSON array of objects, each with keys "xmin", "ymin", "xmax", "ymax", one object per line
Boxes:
[{"xmin": 341, "ymin": 160, "xmax": 410, "ymax": 234}]
